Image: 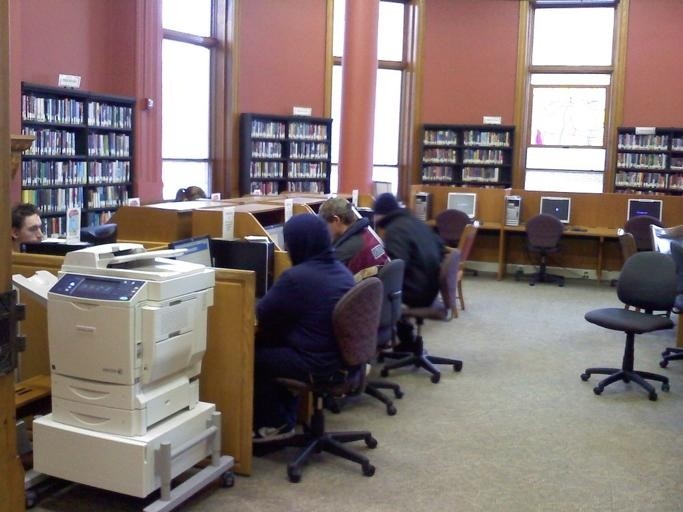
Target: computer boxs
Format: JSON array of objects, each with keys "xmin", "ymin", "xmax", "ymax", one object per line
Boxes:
[
  {"xmin": 414, "ymin": 191, "xmax": 432, "ymax": 221},
  {"xmin": 358, "ymin": 209, "xmax": 380, "ymax": 234},
  {"xmin": 21, "ymin": 241, "xmax": 84, "ymax": 253},
  {"xmin": 212, "ymin": 238, "xmax": 274, "ymax": 298},
  {"xmin": 505, "ymin": 195, "xmax": 521, "ymax": 226}
]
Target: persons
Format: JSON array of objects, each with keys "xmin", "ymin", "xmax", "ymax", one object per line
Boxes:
[
  {"xmin": 174, "ymin": 186, "xmax": 206, "ymax": 201},
  {"xmin": 250, "ymin": 212, "xmax": 356, "ymax": 444},
  {"xmin": 11, "ymin": 203, "xmax": 43, "ymax": 252},
  {"xmin": 372, "ymin": 192, "xmax": 443, "ymax": 307},
  {"xmin": 320, "ymin": 197, "xmax": 388, "ymax": 273}
]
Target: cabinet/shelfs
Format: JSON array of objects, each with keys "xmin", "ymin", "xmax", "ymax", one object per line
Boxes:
[
  {"xmin": 239, "ymin": 114, "xmax": 332, "ymax": 195},
  {"xmin": 614, "ymin": 128, "xmax": 682, "ymax": 195},
  {"xmin": 420, "ymin": 123, "xmax": 512, "ymax": 187},
  {"xmin": 23, "ymin": 84, "xmax": 134, "ymax": 237}
]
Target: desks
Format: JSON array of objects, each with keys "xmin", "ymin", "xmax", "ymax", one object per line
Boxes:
[
  {"xmin": 604, "ymin": 193, "xmax": 683, "ymax": 281},
  {"xmin": 497, "ymin": 190, "xmax": 602, "ymax": 284},
  {"xmin": 415, "ymin": 186, "xmax": 505, "ymax": 276}
]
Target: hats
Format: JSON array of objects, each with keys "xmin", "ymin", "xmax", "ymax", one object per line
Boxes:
[{"xmin": 374, "ymin": 193, "xmax": 397, "ymax": 214}]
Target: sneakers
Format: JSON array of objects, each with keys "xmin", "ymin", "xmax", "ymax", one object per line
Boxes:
[{"xmin": 251, "ymin": 422, "xmax": 294, "ymax": 442}]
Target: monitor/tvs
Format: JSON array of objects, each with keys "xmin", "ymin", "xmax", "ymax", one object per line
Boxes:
[
  {"xmin": 538, "ymin": 196, "xmax": 572, "ymax": 225},
  {"xmin": 169, "ymin": 236, "xmax": 214, "ymax": 268},
  {"xmin": 80, "ymin": 223, "xmax": 118, "ymax": 244},
  {"xmin": 446, "ymin": 192, "xmax": 477, "ymax": 220},
  {"xmin": 265, "ymin": 223, "xmax": 286, "ymax": 252},
  {"xmin": 626, "ymin": 199, "xmax": 663, "ymax": 224}
]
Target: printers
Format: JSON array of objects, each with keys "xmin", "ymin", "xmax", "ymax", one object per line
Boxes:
[{"xmin": 12, "ymin": 243, "xmax": 215, "ymax": 435}]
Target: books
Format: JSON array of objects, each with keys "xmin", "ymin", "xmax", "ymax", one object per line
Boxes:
[
  {"xmin": 20, "ymin": 94, "xmax": 131, "ymax": 239},
  {"xmin": 421, "ymin": 129, "xmax": 509, "ymax": 189},
  {"xmin": 613, "ymin": 134, "xmax": 682, "ymax": 196},
  {"xmin": 249, "ymin": 120, "xmax": 328, "ymax": 196}
]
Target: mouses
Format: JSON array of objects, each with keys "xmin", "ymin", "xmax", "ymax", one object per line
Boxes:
[
  {"xmin": 473, "ymin": 221, "xmax": 480, "ymax": 227},
  {"xmin": 616, "ymin": 227, "xmax": 624, "ymax": 235}
]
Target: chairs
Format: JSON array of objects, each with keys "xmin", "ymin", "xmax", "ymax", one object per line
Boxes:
[
  {"xmin": 318, "ymin": 259, "xmax": 404, "ymax": 416},
  {"xmin": 252, "ymin": 277, "xmax": 382, "ymax": 482},
  {"xmin": 581, "ymin": 214, "xmax": 682, "ymax": 403},
  {"xmin": 375, "ymin": 249, "xmax": 462, "ymax": 383},
  {"xmin": 526, "ymin": 214, "xmax": 565, "ymax": 288},
  {"xmin": 435, "ymin": 210, "xmax": 475, "ymax": 274},
  {"xmin": 448, "ymin": 223, "xmax": 475, "ymax": 317}
]
